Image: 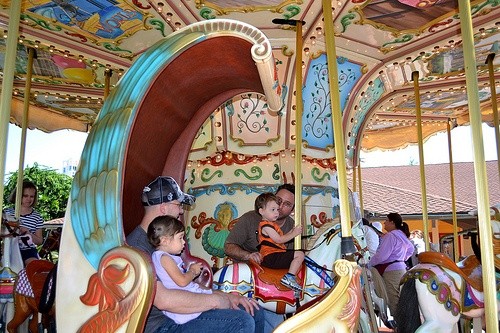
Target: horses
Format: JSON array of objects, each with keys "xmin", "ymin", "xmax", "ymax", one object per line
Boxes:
[
  {"xmin": 0, "ymin": 210, "xmax": 62, "ymax": 333},
  {"xmin": 213, "ymin": 218, "xmax": 371, "ymax": 314},
  {"xmin": 395, "ymin": 206, "xmax": 500, "ymax": 333},
  {"xmin": 361, "ymin": 218, "xmax": 430, "ymax": 265}
]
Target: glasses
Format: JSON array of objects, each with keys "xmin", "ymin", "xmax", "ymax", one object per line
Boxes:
[{"xmin": 277, "ymin": 196, "xmax": 293, "ymax": 209}]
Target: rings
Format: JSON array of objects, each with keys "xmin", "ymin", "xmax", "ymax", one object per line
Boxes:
[{"xmin": 248, "ymin": 299, "xmax": 251, "ymax": 302}]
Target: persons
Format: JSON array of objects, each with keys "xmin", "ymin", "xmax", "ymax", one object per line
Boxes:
[
  {"xmin": 125, "ymin": 175, "xmax": 430, "ymax": 333},
  {"xmin": 3, "ymin": 180, "xmax": 43, "ymax": 268}
]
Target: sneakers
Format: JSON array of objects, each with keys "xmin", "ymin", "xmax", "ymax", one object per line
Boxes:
[{"xmin": 280, "ymin": 274, "xmax": 301, "ymax": 290}]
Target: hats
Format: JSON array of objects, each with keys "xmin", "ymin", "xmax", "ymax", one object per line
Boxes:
[
  {"xmin": 141, "ymin": 175, "xmax": 196, "ymax": 207},
  {"xmin": 371, "ymin": 221, "xmax": 383, "ymax": 232}
]
